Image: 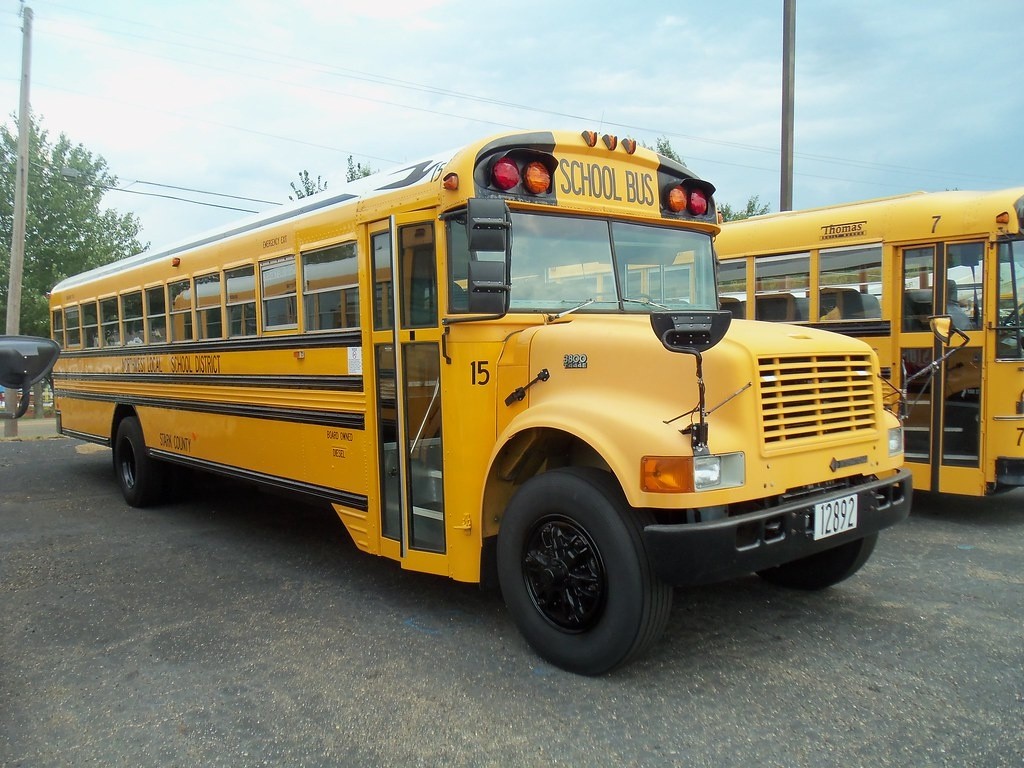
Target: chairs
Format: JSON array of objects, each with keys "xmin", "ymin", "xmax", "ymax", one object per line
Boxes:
[{"xmin": 719, "ymin": 280, "xmax": 983, "ymax": 397}]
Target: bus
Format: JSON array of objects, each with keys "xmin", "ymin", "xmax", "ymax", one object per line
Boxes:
[
  {"xmin": 51, "ymin": 129, "xmax": 971, "ymax": 678},
  {"xmin": 171, "ymin": 250, "xmax": 444, "ymax": 465},
  {"xmin": 457, "ymin": 189, "xmax": 1024, "ymax": 497}
]
